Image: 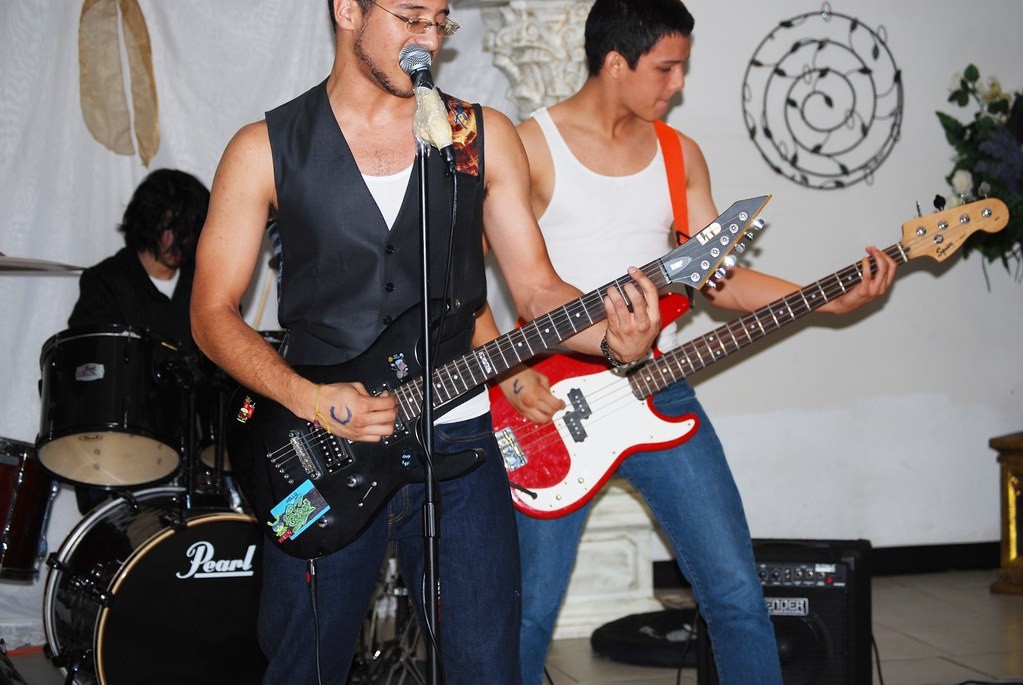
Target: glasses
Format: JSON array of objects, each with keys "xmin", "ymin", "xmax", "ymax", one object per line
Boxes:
[{"xmin": 369, "ymin": 0, "xmax": 460, "ymax": 37}]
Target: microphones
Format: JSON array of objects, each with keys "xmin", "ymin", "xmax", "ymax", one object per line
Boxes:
[{"xmin": 398, "ymin": 44, "xmax": 457, "ymax": 175}]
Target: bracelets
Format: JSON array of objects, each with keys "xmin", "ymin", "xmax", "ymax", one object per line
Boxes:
[
  {"xmin": 600, "ymin": 335, "xmax": 650, "ymax": 368},
  {"xmin": 315, "ymin": 384, "xmax": 331, "ymax": 434}
]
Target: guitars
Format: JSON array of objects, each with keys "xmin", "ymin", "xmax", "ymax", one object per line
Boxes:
[
  {"xmin": 488, "ymin": 179, "xmax": 1009, "ymax": 522},
  {"xmin": 226, "ymin": 190, "xmax": 776, "ymax": 560}
]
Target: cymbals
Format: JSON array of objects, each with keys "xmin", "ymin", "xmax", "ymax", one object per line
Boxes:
[{"xmin": 254, "ymin": 329, "xmax": 288, "ymax": 344}]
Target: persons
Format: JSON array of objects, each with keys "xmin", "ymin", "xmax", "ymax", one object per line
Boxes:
[
  {"xmin": 66, "ymin": 167, "xmax": 209, "ymax": 515},
  {"xmin": 190, "ymin": 0, "xmax": 661, "ymax": 685},
  {"xmin": 472, "ymin": 0, "xmax": 897, "ymax": 685}
]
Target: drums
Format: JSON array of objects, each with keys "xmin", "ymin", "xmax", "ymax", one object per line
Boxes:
[
  {"xmin": 41, "ymin": 483, "xmax": 271, "ymax": 685},
  {"xmin": 31, "ymin": 319, "xmax": 210, "ymax": 494},
  {"xmin": 0, "ymin": 435, "xmax": 65, "ymax": 586}
]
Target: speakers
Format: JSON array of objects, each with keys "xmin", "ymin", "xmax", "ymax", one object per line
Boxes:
[{"xmin": 698, "ymin": 537, "xmax": 873, "ymax": 685}]
